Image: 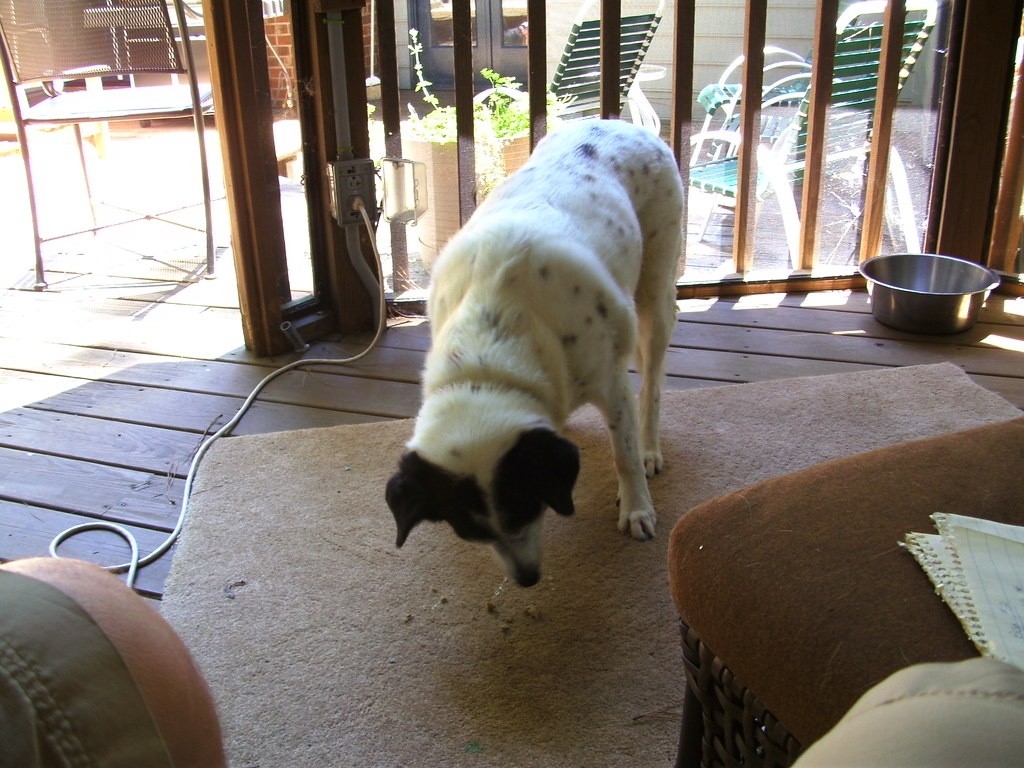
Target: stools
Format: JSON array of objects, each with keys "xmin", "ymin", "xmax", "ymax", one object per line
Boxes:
[{"xmin": 664, "ymin": 417, "xmax": 1024, "ymax": 768}]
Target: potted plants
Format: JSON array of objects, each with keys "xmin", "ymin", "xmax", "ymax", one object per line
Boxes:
[{"xmin": 400, "ymin": 27, "xmax": 579, "ymax": 274}]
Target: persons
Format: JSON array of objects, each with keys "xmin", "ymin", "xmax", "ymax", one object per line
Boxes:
[
  {"xmin": 504, "ymin": 22, "xmax": 528, "ymax": 46},
  {"xmin": 0, "ymin": 555, "xmax": 228, "ymax": 768}
]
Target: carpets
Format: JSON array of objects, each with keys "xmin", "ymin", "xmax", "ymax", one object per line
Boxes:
[{"xmin": 156, "ymin": 360, "xmax": 1024, "ymax": 768}]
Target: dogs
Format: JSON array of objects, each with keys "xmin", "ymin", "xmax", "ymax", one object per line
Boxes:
[{"xmin": 386, "ymin": 115, "xmax": 685, "ymax": 590}]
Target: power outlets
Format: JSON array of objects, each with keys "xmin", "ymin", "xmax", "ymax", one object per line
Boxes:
[{"xmin": 326, "ymin": 157, "xmax": 380, "ymax": 224}]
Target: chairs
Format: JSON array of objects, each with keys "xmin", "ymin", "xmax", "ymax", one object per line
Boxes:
[
  {"xmin": 0, "ymin": 22, "xmax": 115, "ymax": 158},
  {"xmin": 0, "ymin": 0, "xmax": 218, "ymax": 290},
  {"xmin": 689, "ymin": 0, "xmax": 939, "ymax": 272},
  {"xmin": 473, "ymin": 0, "xmax": 666, "ymax": 129}
]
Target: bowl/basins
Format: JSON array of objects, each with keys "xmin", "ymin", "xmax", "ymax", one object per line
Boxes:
[{"xmin": 858, "ymin": 252, "xmax": 1001, "ymax": 335}]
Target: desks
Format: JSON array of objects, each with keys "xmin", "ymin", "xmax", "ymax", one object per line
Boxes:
[{"xmin": 626, "ymin": 61, "xmax": 667, "ymax": 136}]
